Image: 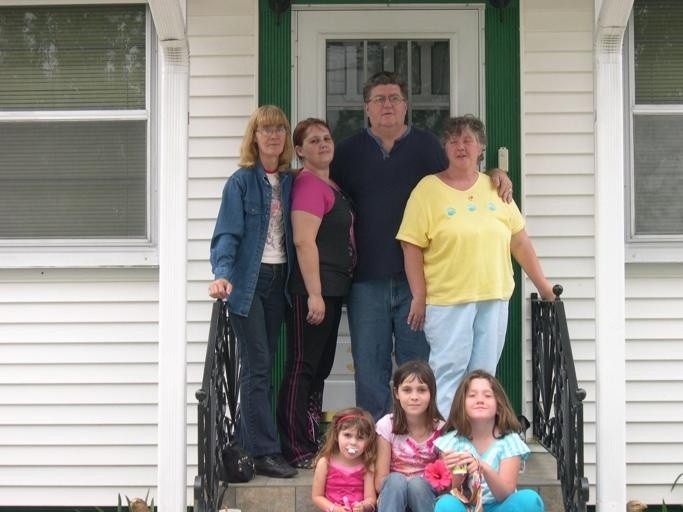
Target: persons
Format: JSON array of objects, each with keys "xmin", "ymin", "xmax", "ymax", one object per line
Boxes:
[
  {"xmin": 207, "ymin": 101, "xmax": 296, "ymax": 482},
  {"xmin": 329, "ymin": 69, "xmax": 514, "ymax": 422},
  {"xmin": 310, "ymin": 405, "xmax": 380, "ymax": 512},
  {"xmin": 394, "ymin": 111, "xmax": 556, "ymax": 416},
  {"xmin": 275, "ymin": 115, "xmax": 361, "ymax": 474},
  {"xmin": 432, "ymin": 368, "xmax": 545, "ymax": 512},
  {"xmin": 375, "ymin": 357, "xmax": 448, "ymax": 512}
]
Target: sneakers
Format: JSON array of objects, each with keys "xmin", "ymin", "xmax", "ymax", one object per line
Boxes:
[
  {"xmin": 254, "ymin": 454, "xmax": 298, "ymax": 478},
  {"xmin": 291, "ymin": 454, "xmax": 321, "ymax": 469}
]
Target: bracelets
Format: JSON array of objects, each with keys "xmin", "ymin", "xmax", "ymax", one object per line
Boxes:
[
  {"xmin": 364, "ymin": 499, "xmax": 376, "ymax": 511},
  {"xmin": 329, "ymin": 502, "xmax": 336, "ymax": 511}
]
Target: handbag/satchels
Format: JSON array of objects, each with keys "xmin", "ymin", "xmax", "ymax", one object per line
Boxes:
[{"xmin": 216, "ymin": 440, "xmax": 256, "ymax": 483}]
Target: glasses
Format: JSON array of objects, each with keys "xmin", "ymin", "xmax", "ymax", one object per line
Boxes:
[
  {"xmin": 365, "ymin": 94, "xmax": 408, "ymax": 106},
  {"xmin": 254, "ymin": 126, "xmax": 288, "ymax": 137}
]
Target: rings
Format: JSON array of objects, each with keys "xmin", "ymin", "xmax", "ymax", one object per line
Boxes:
[{"xmin": 509, "ymin": 188, "xmax": 513, "ymax": 193}]
